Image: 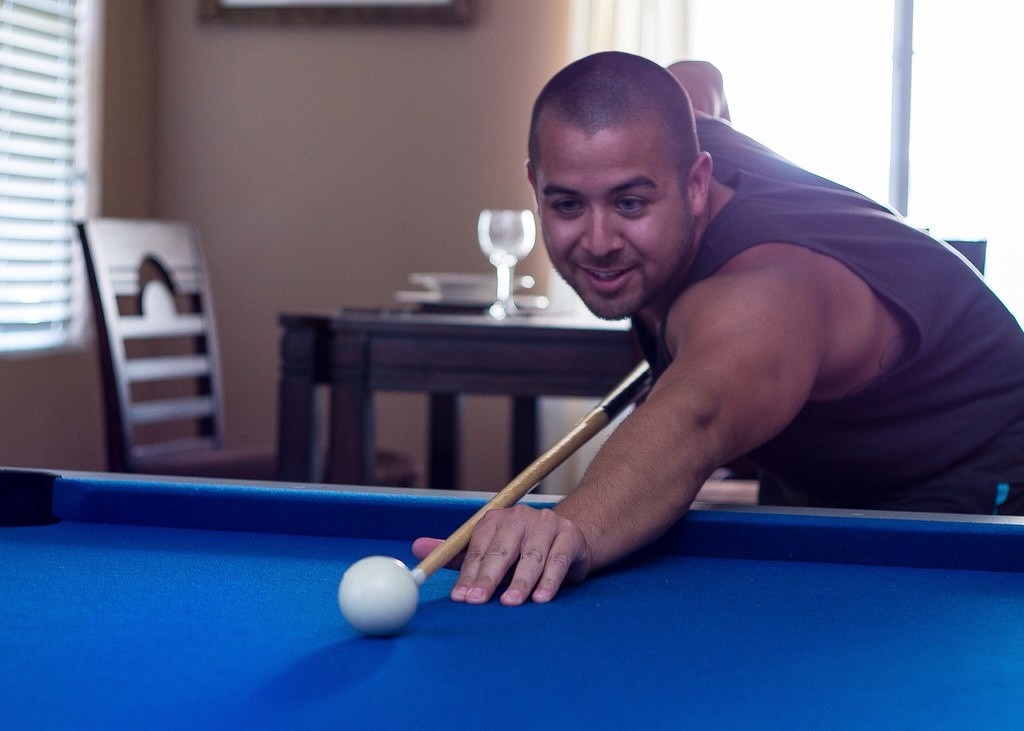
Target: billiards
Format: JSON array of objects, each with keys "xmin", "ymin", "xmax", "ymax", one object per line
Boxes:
[{"xmin": 337, "ymin": 555, "xmax": 417, "ymax": 634}]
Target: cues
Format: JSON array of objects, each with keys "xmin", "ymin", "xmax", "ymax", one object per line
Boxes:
[{"xmin": 404, "ymin": 356, "xmax": 657, "ymax": 583}]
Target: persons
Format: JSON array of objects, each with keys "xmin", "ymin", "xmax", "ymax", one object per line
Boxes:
[{"xmin": 409, "ymin": 48, "xmax": 1023, "ymax": 606}]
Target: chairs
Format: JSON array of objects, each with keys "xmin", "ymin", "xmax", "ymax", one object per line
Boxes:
[{"xmin": 77, "ymin": 220, "xmax": 420, "ymax": 484}]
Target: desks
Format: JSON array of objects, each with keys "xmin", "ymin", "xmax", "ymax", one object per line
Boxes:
[{"xmin": 272, "ymin": 299, "xmax": 655, "ymax": 493}]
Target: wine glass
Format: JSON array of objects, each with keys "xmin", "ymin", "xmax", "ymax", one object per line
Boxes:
[{"xmin": 477, "ymin": 207, "xmax": 537, "ymax": 319}]
